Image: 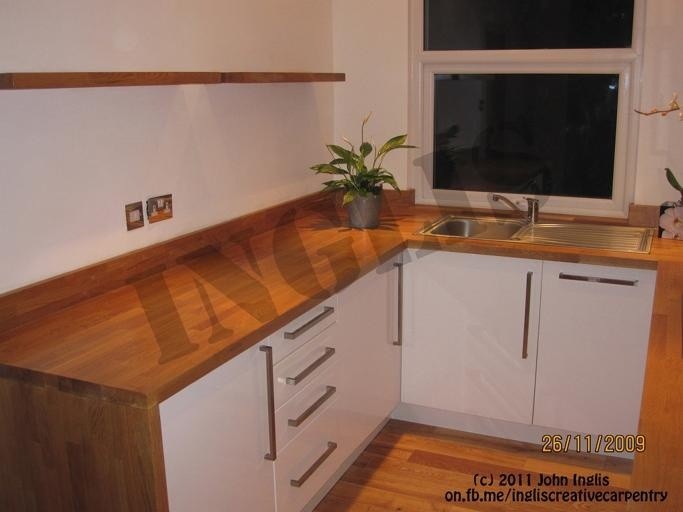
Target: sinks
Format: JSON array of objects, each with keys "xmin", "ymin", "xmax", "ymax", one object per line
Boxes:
[{"xmin": 419, "ymin": 212, "xmax": 529, "ymax": 243}]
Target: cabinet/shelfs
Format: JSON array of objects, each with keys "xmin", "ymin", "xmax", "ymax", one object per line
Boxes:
[{"xmin": 157, "ymin": 246, "xmax": 657, "ymax": 512}]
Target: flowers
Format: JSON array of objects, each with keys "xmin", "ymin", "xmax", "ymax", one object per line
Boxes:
[
  {"xmin": 308, "ymin": 109, "xmax": 421, "ymax": 206},
  {"xmin": 633, "ymin": 92, "xmax": 683, "ymax": 123}
]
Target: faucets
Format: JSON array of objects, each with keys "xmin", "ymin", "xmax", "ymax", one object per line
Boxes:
[{"xmin": 491, "ymin": 193, "xmax": 539, "ymax": 225}]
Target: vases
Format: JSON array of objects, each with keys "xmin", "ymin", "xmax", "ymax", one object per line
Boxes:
[{"xmin": 348, "ymin": 191, "xmax": 381, "ymax": 229}]
